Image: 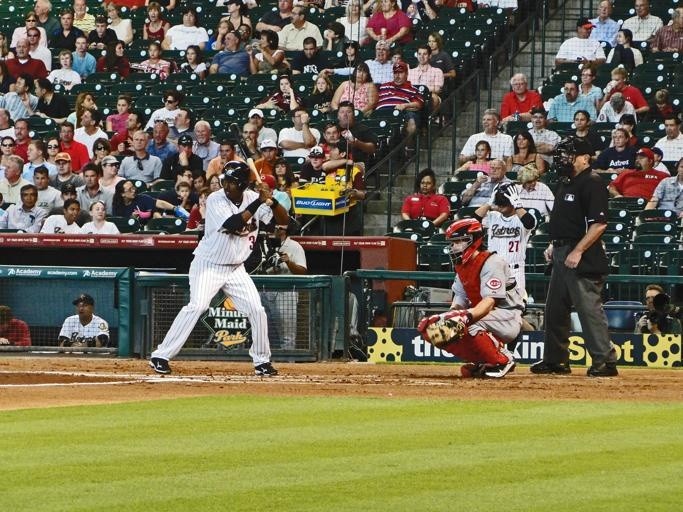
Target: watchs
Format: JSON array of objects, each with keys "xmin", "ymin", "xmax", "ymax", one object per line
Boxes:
[{"xmin": 146, "ymin": 161, "xmax": 291, "ymax": 374}]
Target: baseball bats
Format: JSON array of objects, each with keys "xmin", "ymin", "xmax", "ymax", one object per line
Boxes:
[{"xmin": 229, "ymin": 123, "xmax": 273, "ymax": 207}]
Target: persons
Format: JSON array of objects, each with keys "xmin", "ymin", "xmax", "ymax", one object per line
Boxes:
[
  {"xmin": 530, "ymin": 135, "xmax": 617, "ymax": 375},
  {"xmin": 634, "ymin": 285, "xmax": 681, "ymax": 334},
  {"xmin": 401, "ymin": 1, "xmax": 682, "ymax": 292},
  {"xmin": 261, "ymin": 224, "xmax": 307, "ymax": 350},
  {"xmin": 1, "ymin": 306, "xmax": 32, "ymax": 346},
  {"xmin": 473, "ymin": 182, "xmax": 536, "ymax": 303},
  {"xmin": 1, "ymin": 1, "xmax": 518, "ymax": 235},
  {"xmin": 415, "ymin": 216, "xmax": 527, "ymax": 378},
  {"xmin": 58, "ymin": 295, "xmax": 110, "ymax": 348}
]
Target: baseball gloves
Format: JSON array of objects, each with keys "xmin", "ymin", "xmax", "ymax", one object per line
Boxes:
[{"xmin": 417, "ymin": 309, "xmax": 465, "ymax": 345}]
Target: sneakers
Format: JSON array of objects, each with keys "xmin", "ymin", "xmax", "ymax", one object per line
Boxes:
[
  {"xmin": 587, "ymin": 366, "xmax": 618, "ymax": 376},
  {"xmin": 530, "ymin": 360, "xmax": 570, "ymax": 373},
  {"xmin": 255, "ymin": 362, "xmax": 277, "ymax": 375},
  {"xmin": 149, "ymin": 358, "xmax": 171, "ymax": 373},
  {"xmin": 485, "ymin": 359, "xmax": 515, "ymax": 378}
]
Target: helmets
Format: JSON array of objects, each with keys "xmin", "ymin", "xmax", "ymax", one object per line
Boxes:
[
  {"xmin": 443, "ymin": 218, "xmax": 484, "ymax": 265},
  {"xmin": 494, "ymin": 182, "xmax": 517, "ymax": 205},
  {"xmin": 218, "ymin": 161, "xmax": 250, "ymax": 191},
  {"xmin": 553, "ymin": 135, "xmax": 594, "ymax": 175}
]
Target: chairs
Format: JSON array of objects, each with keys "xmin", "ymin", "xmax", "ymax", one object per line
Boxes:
[
  {"xmin": 382, "ymin": 0, "xmax": 680, "ymax": 276},
  {"xmin": 2, "ymin": 0, "xmax": 554, "ymax": 277}
]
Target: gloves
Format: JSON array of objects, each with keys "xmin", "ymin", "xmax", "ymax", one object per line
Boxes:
[
  {"xmin": 504, "ymin": 185, "xmax": 523, "ymax": 209},
  {"xmin": 487, "ymin": 183, "xmax": 498, "ymax": 205}
]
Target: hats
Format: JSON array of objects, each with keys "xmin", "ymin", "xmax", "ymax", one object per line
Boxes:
[
  {"xmin": 393, "ymin": 62, "xmax": 407, "ymax": 72},
  {"xmin": 62, "ymin": 184, "xmax": 76, "ymax": 193},
  {"xmin": 72, "ymin": 294, "xmax": 94, "ymax": 305},
  {"xmin": 178, "ymin": 135, "xmax": 192, "ymax": 145},
  {"xmin": 55, "ymin": 152, "xmax": 71, "ymax": 161},
  {"xmin": 577, "ymin": 18, "xmax": 596, "ymax": 28},
  {"xmin": 636, "ymin": 147, "xmax": 654, "ymax": 161},
  {"xmin": 101, "ymin": 155, "xmax": 120, "ymax": 165},
  {"xmin": 308, "ymin": 146, "xmax": 324, "ymax": 157},
  {"xmin": 248, "ymin": 108, "xmax": 277, "ymax": 149}
]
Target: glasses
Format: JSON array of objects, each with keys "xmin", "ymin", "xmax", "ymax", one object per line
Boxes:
[
  {"xmin": 3, "ymin": 143, "xmax": 13, "ymax": 146},
  {"xmin": 97, "ymin": 147, "xmax": 105, "ymax": 150},
  {"xmin": 164, "ymin": 100, "xmax": 174, "ymax": 103},
  {"xmin": 48, "ymin": 144, "xmax": 58, "ymax": 148}
]
